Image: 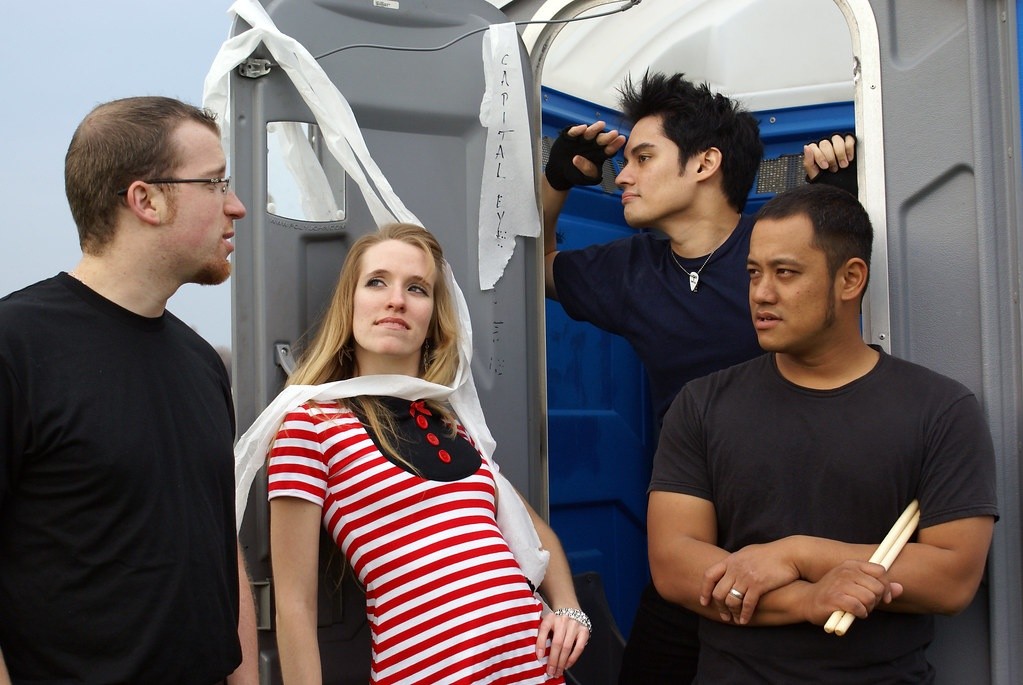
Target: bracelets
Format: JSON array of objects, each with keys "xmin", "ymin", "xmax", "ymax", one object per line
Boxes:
[{"xmin": 552, "ymin": 607, "xmax": 593, "ymax": 634}]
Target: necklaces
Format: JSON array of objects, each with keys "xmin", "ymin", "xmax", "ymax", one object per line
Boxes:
[{"xmin": 671, "ymin": 225, "xmax": 739, "ymax": 292}]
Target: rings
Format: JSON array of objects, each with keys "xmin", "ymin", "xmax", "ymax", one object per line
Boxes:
[{"xmin": 728, "ymin": 588, "xmax": 743, "ymax": 599}]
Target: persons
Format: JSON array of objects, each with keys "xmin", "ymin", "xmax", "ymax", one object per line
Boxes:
[
  {"xmin": 544, "ymin": 73, "xmax": 862, "ymax": 685},
  {"xmin": 646, "ymin": 180, "xmax": 1003, "ymax": 685},
  {"xmin": 0, "ymin": 95, "xmax": 259, "ymax": 685},
  {"xmin": 265, "ymin": 222, "xmax": 594, "ymax": 685}
]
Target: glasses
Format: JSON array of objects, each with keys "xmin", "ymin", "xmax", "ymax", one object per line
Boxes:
[{"xmin": 117, "ymin": 175, "xmax": 232, "ymax": 200}]
